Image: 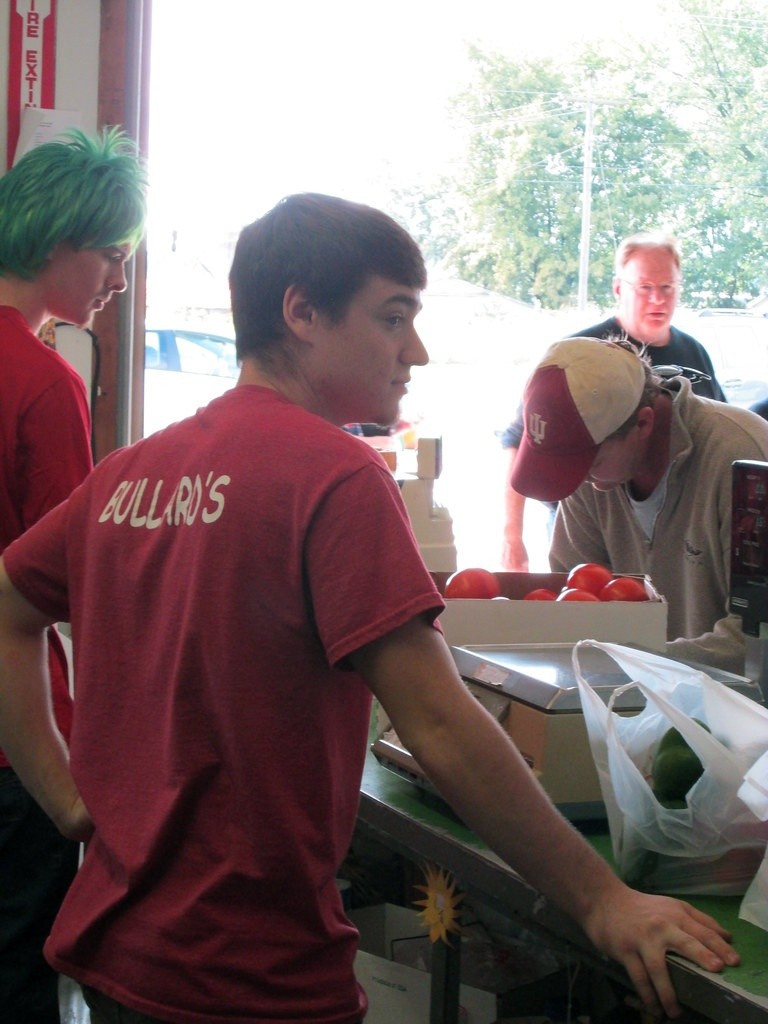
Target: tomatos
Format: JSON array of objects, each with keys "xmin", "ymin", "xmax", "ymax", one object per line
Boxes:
[
  {"xmin": 524, "ymin": 589, "xmax": 556, "ymax": 600},
  {"xmin": 599, "ymin": 578, "xmax": 649, "ymax": 602},
  {"xmin": 567, "ymin": 563, "xmax": 611, "ymax": 593},
  {"xmin": 556, "ymin": 589, "xmax": 599, "ymax": 601},
  {"xmin": 445, "ymin": 568, "xmax": 500, "ymax": 598}
]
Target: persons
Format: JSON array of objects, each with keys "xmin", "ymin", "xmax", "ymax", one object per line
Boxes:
[
  {"xmin": 342, "ymin": 416, "xmax": 415, "ymax": 445},
  {"xmin": 500, "ymin": 228, "xmax": 727, "ymax": 572},
  {"xmin": 0, "ymin": 193, "xmax": 738, "ymax": 1024},
  {"xmin": 511, "ymin": 336, "xmax": 768, "ymax": 677},
  {"xmin": 0, "ymin": 130, "xmax": 149, "ymax": 1024}
]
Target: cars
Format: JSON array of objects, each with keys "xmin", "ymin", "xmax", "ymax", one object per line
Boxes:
[{"xmin": 141, "ymin": 326, "xmax": 419, "ymax": 467}]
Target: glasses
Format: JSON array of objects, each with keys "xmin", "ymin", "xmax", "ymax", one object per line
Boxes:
[{"xmin": 620, "ymin": 276, "xmax": 681, "ymax": 297}]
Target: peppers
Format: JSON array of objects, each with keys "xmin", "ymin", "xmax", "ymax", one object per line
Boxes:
[
  {"xmin": 623, "ymin": 784, "xmax": 685, "ymax": 884},
  {"xmin": 651, "ymin": 717, "xmax": 712, "ymax": 797}
]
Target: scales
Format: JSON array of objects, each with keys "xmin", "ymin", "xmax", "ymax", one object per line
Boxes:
[{"xmin": 372, "ymin": 638, "xmax": 764, "ymax": 825}]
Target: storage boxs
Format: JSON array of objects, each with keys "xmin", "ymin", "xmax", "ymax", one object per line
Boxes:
[{"xmin": 425, "ymin": 568, "xmax": 669, "ymax": 646}]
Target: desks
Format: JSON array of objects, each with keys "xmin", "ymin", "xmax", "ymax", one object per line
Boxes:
[{"xmin": 353, "ymin": 699, "xmax": 768, "ymax": 1024}]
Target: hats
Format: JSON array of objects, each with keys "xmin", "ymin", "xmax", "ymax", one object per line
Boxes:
[{"xmin": 511, "ymin": 336, "xmax": 646, "ymax": 500}]
